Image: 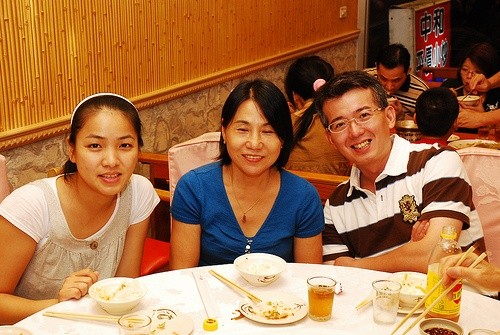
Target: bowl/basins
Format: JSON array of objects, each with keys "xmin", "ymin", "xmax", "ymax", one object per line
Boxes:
[
  {"xmin": 88, "ymin": 277, "xmax": 145, "ymax": 315},
  {"xmin": 418, "ymin": 317, "xmax": 465, "ymax": 335},
  {"xmin": 468, "ymin": 329, "xmax": 496, "ymax": 335},
  {"xmin": 389, "ymin": 271, "xmax": 428, "ymax": 307},
  {"xmin": 0, "ymin": 325, "xmax": 31, "ymax": 335},
  {"xmin": 386, "ymin": 98, "xmax": 398, "ymax": 103},
  {"xmin": 457, "ymin": 95, "xmax": 481, "ymax": 105},
  {"xmin": 234, "ymin": 252, "xmax": 287, "ymax": 287}
]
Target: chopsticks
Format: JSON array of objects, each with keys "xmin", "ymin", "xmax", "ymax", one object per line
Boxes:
[
  {"xmin": 459, "ymin": 90, "xmax": 473, "ymax": 104},
  {"xmin": 44, "ymin": 311, "xmax": 144, "ymax": 322},
  {"xmin": 355, "ymin": 297, "xmax": 372, "ymax": 310},
  {"xmin": 391, "ymin": 246, "xmax": 487, "ymax": 335},
  {"xmin": 209, "ymin": 269, "xmax": 262, "ymax": 304}
]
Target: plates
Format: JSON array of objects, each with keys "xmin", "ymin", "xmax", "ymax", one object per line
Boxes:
[
  {"xmin": 448, "ymin": 139, "xmax": 498, "ymax": 150},
  {"xmin": 396, "ymin": 120, "xmax": 419, "ymax": 131},
  {"xmin": 136, "ymin": 308, "xmax": 193, "ymax": 335},
  {"xmin": 447, "ymin": 134, "xmax": 460, "ymax": 141},
  {"xmin": 238, "ymin": 293, "xmax": 308, "ymax": 324},
  {"xmin": 398, "ymin": 307, "xmax": 425, "ymax": 314}
]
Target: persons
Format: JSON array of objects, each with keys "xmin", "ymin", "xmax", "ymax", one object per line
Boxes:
[
  {"xmin": 170, "ymin": 79, "xmax": 324, "ymax": 272},
  {"xmin": 316, "ymin": 71, "xmax": 500, "ymax": 300},
  {"xmin": 360, "ymin": 44, "xmax": 430, "ymax": 121},
  {"xmin": 0, "ymin": 93, "xmax": 161, "ymax": 326},
  {"xmin": 284, "ymin": 55, "xmax": 351, "ymax": 177},
  {"xmin": 439, "ymin": 44, "xmax": 500, "ymax": 134},
  {"xmin": 438, "ymin": 252, "xmax": 500, "ymax": 301}
]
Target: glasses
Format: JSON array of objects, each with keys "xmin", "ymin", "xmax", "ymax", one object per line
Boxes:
[
  {"xmin": 327, "ymin": 107, "xmax": 385, "ymax": 134},
  {"xmin": 459, "ymin": 68, "xmax": 480, "ymax": 77}
]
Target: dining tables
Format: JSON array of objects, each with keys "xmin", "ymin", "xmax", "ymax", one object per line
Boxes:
[{"xmin": 15, "ymin": 262, "xmax": 500, "ymax": 335}]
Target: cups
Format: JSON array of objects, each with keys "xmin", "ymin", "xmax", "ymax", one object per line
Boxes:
[
  {"xmin": 118, "ymin": 313, "xmax": 151, "ymax": 335},
  {"xmin": 372, "ymin": 280, "xmax": 402, "ymax": 324},
  {"xmin": 307, "ymin": 276, "xmax": 337, "ymax": 322}
]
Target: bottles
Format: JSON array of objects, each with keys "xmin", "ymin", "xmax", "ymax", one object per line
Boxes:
[{"xmin": 425, "ymin": 224, "xmax": 463, "ymax": 321}]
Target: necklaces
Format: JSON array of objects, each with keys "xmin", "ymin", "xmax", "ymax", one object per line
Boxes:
[{"xmin": 228, "ymin": 165, "xmax": 272, "ymax": 223}]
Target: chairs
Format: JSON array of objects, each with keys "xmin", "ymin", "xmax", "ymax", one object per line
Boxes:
[{"xmin": 288, "ymin": 169, "xmax": 350, "ymax": 206}]
